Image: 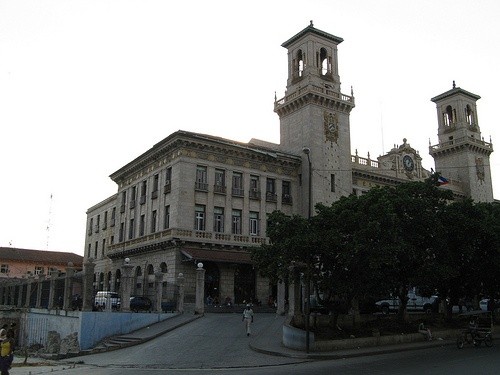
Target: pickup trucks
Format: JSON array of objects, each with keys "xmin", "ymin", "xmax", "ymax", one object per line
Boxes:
[{"xmin": 374, "ymin": 286, "xmax": 451, "ymax": 314}]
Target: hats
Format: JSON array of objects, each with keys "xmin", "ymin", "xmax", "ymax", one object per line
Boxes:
[{"xmin": 246, "ymin": 304, "xmax": 250, "ymax": 306}]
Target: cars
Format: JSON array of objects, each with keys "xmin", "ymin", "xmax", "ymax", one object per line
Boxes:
[
  {"xmin": 160, "ymin": 298, "xmax": 178, "ymax": 314},
  {"xmin": 57, "ymin": 294, "xmax": 82, "ymax": 310},
  {"xmin": 477, "ymin": 299, "xmax": 496, "ymax": 311},
  {"xmin": 116, "ymin": 296, "xmax": 152, "ymax": 312}
]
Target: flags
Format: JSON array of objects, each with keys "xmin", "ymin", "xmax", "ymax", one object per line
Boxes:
[{"xmin": 436, "ymin": 177, "xmax": 448, "ymax": 186}]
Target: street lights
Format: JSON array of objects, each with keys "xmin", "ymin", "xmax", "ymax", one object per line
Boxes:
[{"xmin": 302, "ymin": 146, "xmax": 313, "ymax": 353}]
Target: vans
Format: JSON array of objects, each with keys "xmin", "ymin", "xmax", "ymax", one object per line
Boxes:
[{"xmin": 94, "ymin": 291, "xmax": 121, "ymax": 309}]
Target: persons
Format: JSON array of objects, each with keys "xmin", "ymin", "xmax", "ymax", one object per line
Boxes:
[
  {"xmin": 468, "ymin": 317, "xmax": 475, "ymax": 338},
  {"xmin": 241, "ymin": 304, "xmax": 254, "ymax": 336},
  {"xmin": 417, "ymin": 320, "xmax": 432, "ymax": 339},
  {"xmin": 487, "ymin": 297, "xmax": 497, "ymax": 324},
  {"xmin": 206, "ymin": 295, "xmax": 232, "ymax": 312},
  {"xmin": 269, "ymin": 295, "xmax": 287, "ymax": 309},
  {"xmin": 0, "ymin": 329, "xmax": 16, "ymax": 375}
]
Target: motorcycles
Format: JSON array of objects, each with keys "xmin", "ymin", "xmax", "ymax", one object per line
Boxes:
[{"xmin": 457, "ymin": 317, "xmax": 493, "ymax": 349}]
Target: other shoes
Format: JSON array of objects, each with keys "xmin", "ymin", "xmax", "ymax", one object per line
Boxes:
[{"xmin": 247, "ymin": 332, "xmax": 250, "ymax": 336}]
